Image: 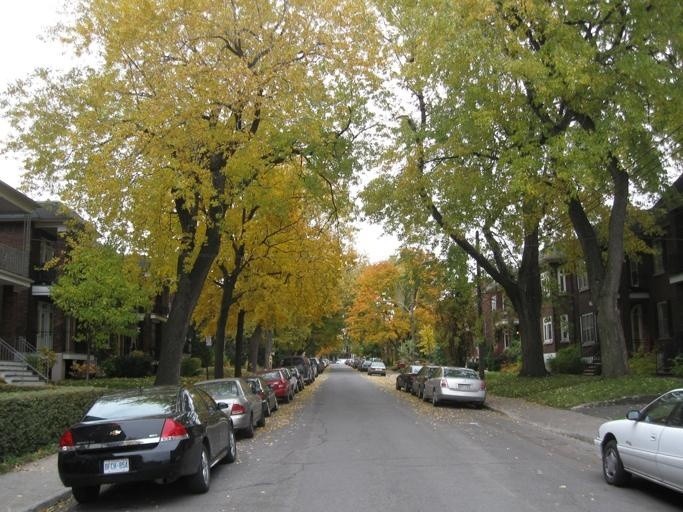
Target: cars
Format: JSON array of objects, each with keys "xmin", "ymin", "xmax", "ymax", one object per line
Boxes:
[
  {"xmin": 396, "ymin": 364, "xmax": 486, "ymax": 407},
  {"xmin": 244, "ymin": 376, "xmax": 278, "ymax": 417},
  {"xmin": 279, "ymin": 355, "xmax": 329, "ymax": 391},
  {"xmin": 345, "ymin": 357, "xmax": 386, "ymax": 376},
  {"xmin": 256, "ymin": 367, "xmax": 299, "ymax": 403},
  {"xmin": 58, "ymin": 382, "xmax": 236, "ymax": 504},
  {"xmin": 594, "ymin": 388, "xmax": 683, "ymax": 494},
  {"xmin": 192, "ymin": 377, "xmax": 265, "ymax": 438}
]
